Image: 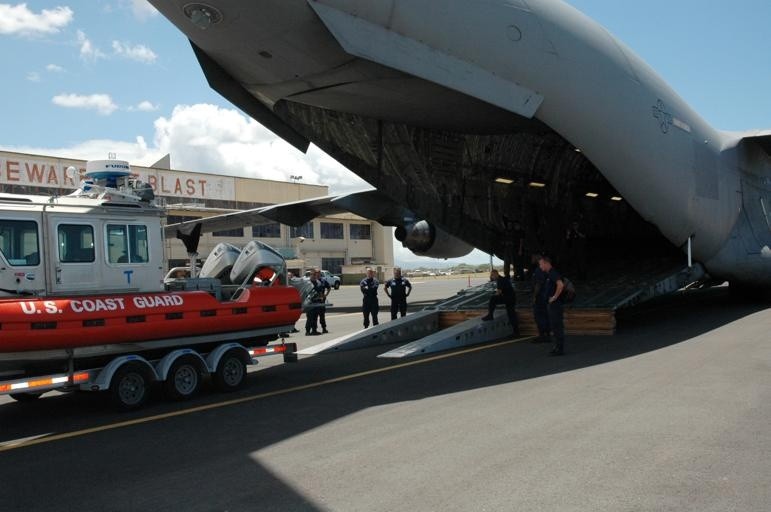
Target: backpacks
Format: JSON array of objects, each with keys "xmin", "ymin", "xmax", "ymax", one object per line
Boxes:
[{"xmin": 559, "ymin": 276, "xmax": 576, "ymax": 304}]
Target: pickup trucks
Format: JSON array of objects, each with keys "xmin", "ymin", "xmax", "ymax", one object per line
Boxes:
[{"xmin": 301, "ymin": 270, "xmax": 341, "ymax": 289}]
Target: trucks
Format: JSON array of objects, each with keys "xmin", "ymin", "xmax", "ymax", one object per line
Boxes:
[{"xmin": 0, "ymin": 160, "xmax": 333, "ymax": 413}]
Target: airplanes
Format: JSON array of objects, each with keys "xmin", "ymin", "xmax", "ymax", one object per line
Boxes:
[{"xmin": 109, "ymin": 0, "xmax": 771, "ymax": 309}]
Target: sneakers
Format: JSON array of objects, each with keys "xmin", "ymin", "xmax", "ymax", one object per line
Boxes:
[
  {"xmin": 507, "ymin": 331, "xmax": 519, "ymax": 339},
  {"xmin": 529, "ymin": 336, "xmax": 565, "ymax": 355},
  {"xmin": 279, "ymin": 327, "xmax": 328, "ymax": 340},
  {"xmin": 481, "ymin": 315, "xmax": 495, "ymax": 321}
]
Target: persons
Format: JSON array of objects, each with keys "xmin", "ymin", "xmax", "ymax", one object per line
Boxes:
[
  {"xmin": 539, "ymin": 256, "xmax": 570, "ymax": 356},
  {"xmin": 481, "ymin": 270, "xmax": 521, "ymax": 339},
  {"xmin": 530, "ymin": 251, "xmax": 552, "ymax": 344},
  {"xmin": 288, "ymin": 272, "xmax": 300, "ymax": 332},
  {"xmin": 360, "ymin": 269, "xmax": 380, "ymax": 329},
  {"xmin": 305, "ymin": 270, "xmax": 324, "ymax": 335},
  {"xmin": 384, "ymin": 269, "xmax": 413, "ymax": 320},
  {"xmin": 313, "ymin": 267, "xmax": 332, "ymax": 333}
]
